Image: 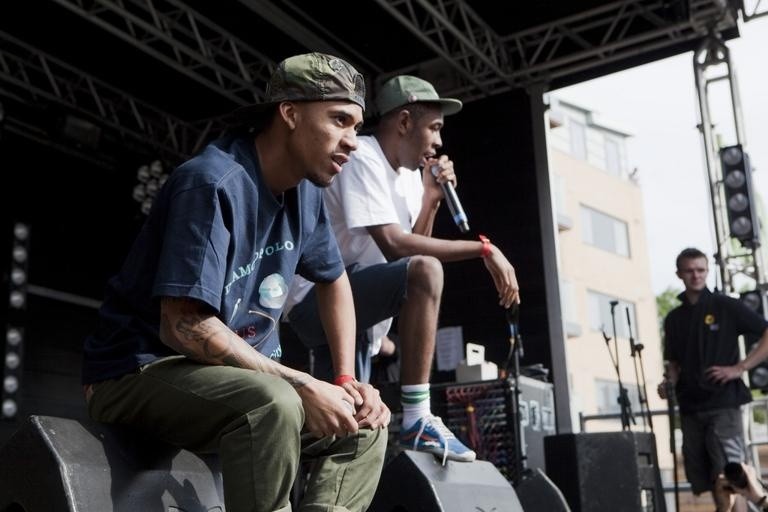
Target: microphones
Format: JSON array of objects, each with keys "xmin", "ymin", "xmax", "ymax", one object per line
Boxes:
[
  {"xmin": 505, "ymin": 300, "xmax": 525, "ymax": 357},
  {"xmin": 431, "ymin": 164, "xmax": 469, "ymax": 233}
]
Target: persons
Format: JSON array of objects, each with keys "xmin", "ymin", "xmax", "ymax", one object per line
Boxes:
[
  {"xmin": 280, "ymin": 74, "xmax": 523, "ymax": 465},
  {"xmin": 372, "ymin": 330, "xmax": 401, "ymax": 383},
  {"xmin": 654, "ymin": 248, "xmax": 767, "ymax": 512},
  {"xmin": 79, "ymin": 50, "xmax": 391, "ymax": 512},
  {"xmin": 709, "ymin": 460, "xmax": 767, "ymax": 512}
]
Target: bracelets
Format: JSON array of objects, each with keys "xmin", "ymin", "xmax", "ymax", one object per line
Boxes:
[
  {"xmin": 333, "ymin": 375, "xmax": 358, "ymax": 387},
  {"xmin": 475, "ymin": 233, "xmax": 492, "ymax": 261},
  {"xmin": 755, "ymin": 496, "xmax": 766, "ymax": 508}
]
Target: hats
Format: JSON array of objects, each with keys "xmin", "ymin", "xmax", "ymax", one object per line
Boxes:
[
  {"xmin": 229, "ymin": 52, "xmax": 367, "ymax": 125},
  {"xmin": 374, "ymin": 74, "xmax": 463, "ymax": 118}
]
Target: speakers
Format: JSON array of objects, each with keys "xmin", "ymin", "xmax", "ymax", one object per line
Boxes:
[
  {"xmin": 366, "ymin": 451, "xmax": 524, "ymax": 512},
  {"xmin": 516, "ymin": 468, "xmax": 572, "ymax": 512},
  {"xmin": 0, "ymin": 415, "xmax": 226, "ymax": 512}
]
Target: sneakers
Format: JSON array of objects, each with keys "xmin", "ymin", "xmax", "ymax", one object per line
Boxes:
[{"xmin": 398, "ymin": 413, "xmax": 478, "ymax": 466}]
